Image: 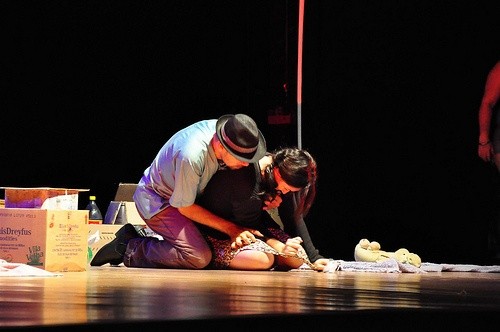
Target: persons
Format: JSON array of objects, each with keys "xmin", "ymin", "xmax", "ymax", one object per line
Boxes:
[
  {"xmin": 192, "ymin": 148, "xmax": 341, "ymax": 272},
  {"xmin": 90, "ymin": 112, "xmax": 267, "ymax": 270},
  {"xmin": 478, "ymin": 62, "xmax": 500, "ymax": 171}
]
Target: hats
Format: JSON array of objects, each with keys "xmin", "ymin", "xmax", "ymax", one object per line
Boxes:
[{"xmin": 216, "ymin": 113, "xmax": 266, "ymax": 163}]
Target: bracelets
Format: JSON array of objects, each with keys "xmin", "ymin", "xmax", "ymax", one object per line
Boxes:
[{"xmin": 478, "ymin": 141, "xmax": 490, "ymax": 146}]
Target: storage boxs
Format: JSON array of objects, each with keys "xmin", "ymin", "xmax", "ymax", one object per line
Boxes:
[{"xmin": 0, "ymin": 184, "xmax": 164, "ymax": 272}]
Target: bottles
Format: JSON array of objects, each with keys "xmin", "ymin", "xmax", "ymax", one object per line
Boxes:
[{"xmin": 83, "ymin": 196, "xmax": 102, "ymax": 225}]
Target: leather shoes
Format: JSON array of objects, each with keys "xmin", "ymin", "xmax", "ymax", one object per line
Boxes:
[{"xmin": 90, "ymin": 223, "xmax": 141, "ymax": 266}]
